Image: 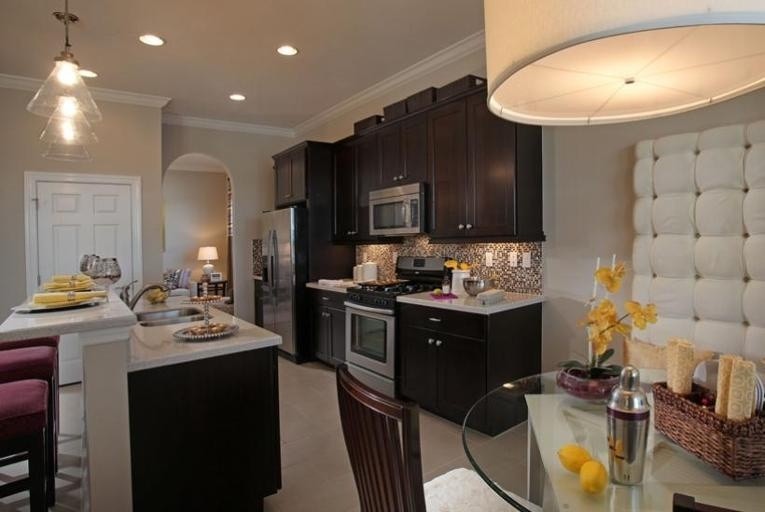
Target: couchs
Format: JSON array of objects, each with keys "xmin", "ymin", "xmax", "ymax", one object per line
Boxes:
[{"xmin": 163, "ymin": 280, "xmax": 198, "ymax": 296}]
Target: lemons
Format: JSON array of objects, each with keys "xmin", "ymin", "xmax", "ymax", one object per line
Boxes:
[
  {"xmin": 580, "ymin": 461, "xmax": 607, "ymax": 496},
  {"xmin": 556, "ymin": 444, "xmax": 592, "ymax": 474}
]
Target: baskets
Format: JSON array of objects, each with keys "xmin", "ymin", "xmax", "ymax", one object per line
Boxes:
[{"xmin": 652, "ymin": 379, "xmax": 765, "ymax": 481}]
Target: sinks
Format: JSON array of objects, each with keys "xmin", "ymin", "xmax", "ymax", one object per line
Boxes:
[
  {"xmin": 136, "ymin": 306, "xmax": 203, "ymax": 321},
  {"xmin": 135, "ymin": 315, "xmax": 214, "ymax": 327}
]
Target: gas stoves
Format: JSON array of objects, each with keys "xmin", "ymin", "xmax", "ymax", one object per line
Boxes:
[{"xmin": 346, "ymin": 274, "xmax": 442, "ymax": 308}]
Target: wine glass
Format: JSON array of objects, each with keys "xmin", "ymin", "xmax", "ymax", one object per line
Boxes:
[{"xmin": 80, "ymin": 254, "xmax": 123, "ymax": 295}]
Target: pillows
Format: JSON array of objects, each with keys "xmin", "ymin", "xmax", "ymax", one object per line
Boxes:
[{"xmin": 173, "ymin": 268, "xmax": 192, "ymax": 288}]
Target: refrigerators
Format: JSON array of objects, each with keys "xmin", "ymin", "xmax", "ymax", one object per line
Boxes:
[{"xmin": 259, "ymin": 205, "xmax": 314, "ymax": 365}]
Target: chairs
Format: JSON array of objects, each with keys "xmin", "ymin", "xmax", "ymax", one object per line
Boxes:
[
  {"xmin": 335, "ymin": 362, "xmax": 543, "ymax": 512},
  {"xmin": 673, "ymin": 492, "xmax": 748, "ymax": 512}
]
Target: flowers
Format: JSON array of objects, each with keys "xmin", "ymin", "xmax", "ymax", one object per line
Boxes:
[{"xmin": 551, "ymin": 258, "xmax": 661, "ymax": 379}]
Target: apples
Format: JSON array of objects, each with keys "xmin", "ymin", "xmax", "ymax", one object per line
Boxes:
[{"xmin": 434, "ymin": 289, "xmax": 442, "ymax": 297}]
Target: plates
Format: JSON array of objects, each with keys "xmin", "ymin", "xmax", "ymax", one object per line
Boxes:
[
  {"xmin": 692, "ymin": 356, "xmax": 765, "ymax": 413},
  {"xmin": 429, "ymin": 293, "xmax": 458, "ymax": 299},
  {"xmin": 42, "ymin": 276, "xmax": 97, "ymax": 292},
  {"xmin": 173, "ymin": 322, "xmax": 239, "ymax": 339},
  {"xmin": 180, "ymin": 294, "xmax": 233, "ymax": 306},
  {"xmin": 9, "ymin": 297, "xmax": 106, "ymax": 313}
]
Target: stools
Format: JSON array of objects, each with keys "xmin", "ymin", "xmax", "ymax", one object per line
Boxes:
[{"xmin": 0, "ymin": 332, "xmax": 60, "ymax": 512}]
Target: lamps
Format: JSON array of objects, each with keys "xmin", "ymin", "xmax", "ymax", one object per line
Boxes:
[
  {"xmin": 24, "ymin": 1, "xmax": 104, "ymax": 164},
  {"xmin": 196, "ymin": 246, "xmax": 219, "ymax": 282},
  {"xmin": 483, "ymin": 0, "xmax": 765, "ymax": 127}
]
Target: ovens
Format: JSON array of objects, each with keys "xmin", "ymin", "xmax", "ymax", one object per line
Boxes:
[{"xmin": 342, "ymin": 299, "xmax": 398, "ymax": 400}]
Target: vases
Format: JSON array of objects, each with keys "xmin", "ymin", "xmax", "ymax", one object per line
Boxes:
[{"xmin": 553, "ymin": 369, "xmax": 620, "ymax": 404}]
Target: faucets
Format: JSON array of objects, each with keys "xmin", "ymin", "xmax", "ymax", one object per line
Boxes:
[{"xmin": 119, "ymin": 279, "xmax": 169, "ymax": 311}]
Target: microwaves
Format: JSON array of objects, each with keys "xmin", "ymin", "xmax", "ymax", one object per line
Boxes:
[{"xmin": 367, "ymin": 181, "xmax": 426, "ymax": 237}]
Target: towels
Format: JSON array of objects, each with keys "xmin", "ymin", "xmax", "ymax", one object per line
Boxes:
[
  {"xmin": 32, "ymin": 273, "xmax": 109, "ymax": 307},
  {"xmin": 714, "ymin": 354, "xmax": 758, "ymax": 423},
  {"xmin": 663, "ymin": 337, "xmax": 695, "ymax": 395}
]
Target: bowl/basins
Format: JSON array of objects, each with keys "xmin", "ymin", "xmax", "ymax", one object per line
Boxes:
[{"xmin": 462, "ymin": 276, "xmax": 502, "ymax": 296}]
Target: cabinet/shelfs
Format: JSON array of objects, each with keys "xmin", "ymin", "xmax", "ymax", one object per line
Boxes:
[
  {"xmin": 396, "ymin": 291, "xmax": 543, "ymax": 439},
  {"xmin": 255, "ymin": 279, "xmax": 263, "ymax": 327},
  {"xmin": 330, "ymin": 73, "xmax": 546, "ymax": 246},
  {"xmin": 306, "ymin": 279, "xmax": 352, "ymax": 369},
  {"xmin": 271, "ymin": 139, "xmax": 334, "ymax": 209}
]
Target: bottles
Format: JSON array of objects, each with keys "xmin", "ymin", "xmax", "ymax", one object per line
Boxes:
[
  {"xmin": 442, "ymin": 275, "xmax": 452, "ymax": 296},
  {"xmin": 605, "ymin": 480, "xmax": 645, "ymax": 512},
  {"xmin": 451, "ymin": 269, "xmax": 471, "ymax": 296},
  {"xmin": 353, "ymin": 259, "xmax": 378, "ymax": 284},
  {"xmin": 606, "ymin": 365, "xmax": 651, "ymax": 486}
]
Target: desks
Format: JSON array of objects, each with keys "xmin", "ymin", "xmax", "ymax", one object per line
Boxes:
[{"xmin": 197, "ymin": 280, "xmax": 229, "ymax": 296}]
